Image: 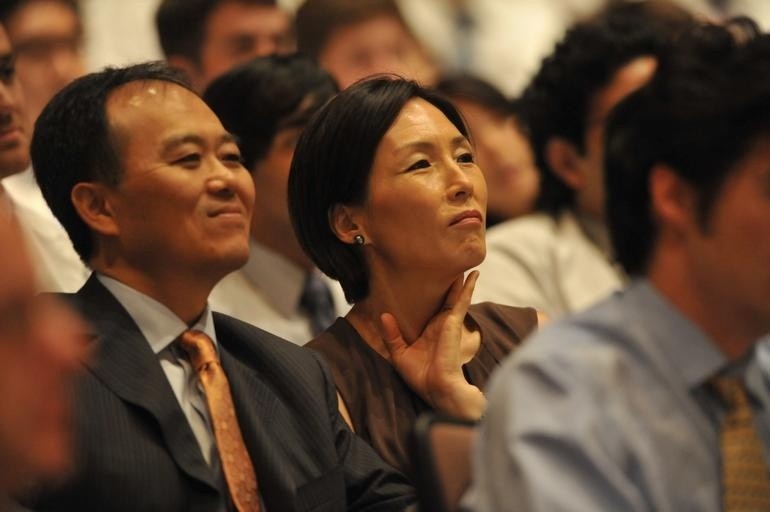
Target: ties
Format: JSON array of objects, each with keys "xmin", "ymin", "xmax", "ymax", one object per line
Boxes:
[
  {"xmin": 175, "ymin": 330, "xmax": 264, "ymax": 512},
  {"xmin": 705, "ymin": 372, "xmax": 770, "ymax": 511}
]
